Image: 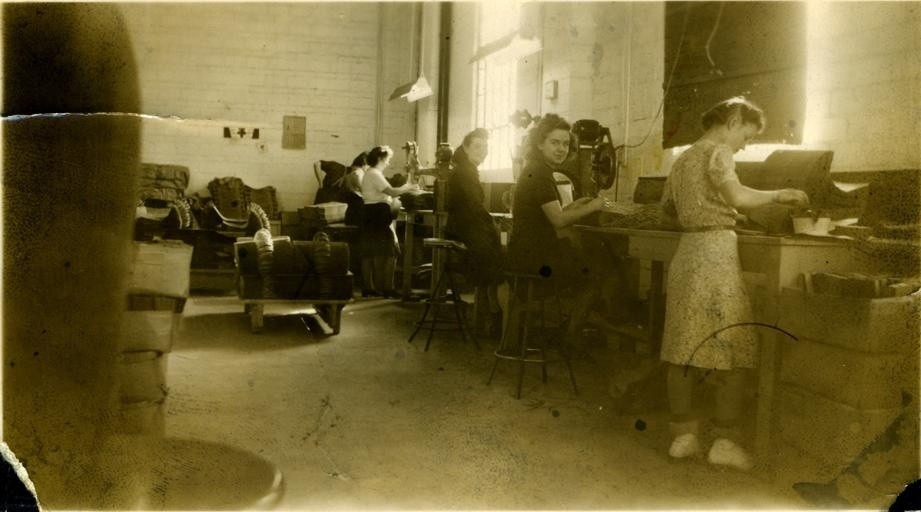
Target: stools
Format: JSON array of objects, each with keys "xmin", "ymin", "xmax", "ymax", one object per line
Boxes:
[
  {"xmin": 486, "ymin": 268, "xmax": 577, "ymax": 398},
  {"xmin": 408, "ymin": 238, "xmax": 483, "ymax": 353}
]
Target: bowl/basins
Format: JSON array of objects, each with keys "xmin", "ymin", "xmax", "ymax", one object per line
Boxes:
[{"xmin": 794, "ymin": 217, "xmax": 830, "ymax": 238}]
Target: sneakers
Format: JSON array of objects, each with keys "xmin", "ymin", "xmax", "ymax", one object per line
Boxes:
[
  {"xmin": 568, "ymin": 322, "xmax": 600, "ymax": 337},
  {"xmin": 669, "ymin": 431, "xmax": 699, "ymax": 457},
  {"xmin": 707, "ymin": 439, "xmax": 754, "ymax": 465}
]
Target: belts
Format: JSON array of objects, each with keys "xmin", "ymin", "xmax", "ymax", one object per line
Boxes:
[{"xmin": 684, "ymin": 224, "xmax": 733, "ymax": 232}]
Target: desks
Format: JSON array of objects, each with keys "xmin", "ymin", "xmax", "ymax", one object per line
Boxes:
[
  {"xmin": 438, "ymin": 212, "xmax": 512, "ymax": 326},
  {"xmin": 627, "ymin": 228, "xmax": 854, "ymax": 472},
  {"xmin": 393, "ymin": 206, "xmax": 437, "ymax": 295},
  {"xmin": 550, "ymin": 222, "xmax": 649, "ymax": 339}
]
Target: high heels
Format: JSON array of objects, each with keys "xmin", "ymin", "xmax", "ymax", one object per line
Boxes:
[{"xmin": 360, "ymin": 287, "xmax": 402, "ymax": 299}]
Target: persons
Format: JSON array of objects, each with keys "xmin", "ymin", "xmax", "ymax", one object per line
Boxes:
[
  {"xmin": 347, "ymin": 151, "xmax": 370, "ymax": 197},
  {"xmin": 446, "ymin": 128, "xmax": 505, "ymax": 339},
  {"xmin": 360, "ymin": 145, "xmax": 419, "ymax": 299},
  {"xmin": 509, "ymin": 115, "xmax": 610, "ymax": 352},
  {"xmin": 659, "ymin": 97, "xmax": 810, "ymax": 469}
]
